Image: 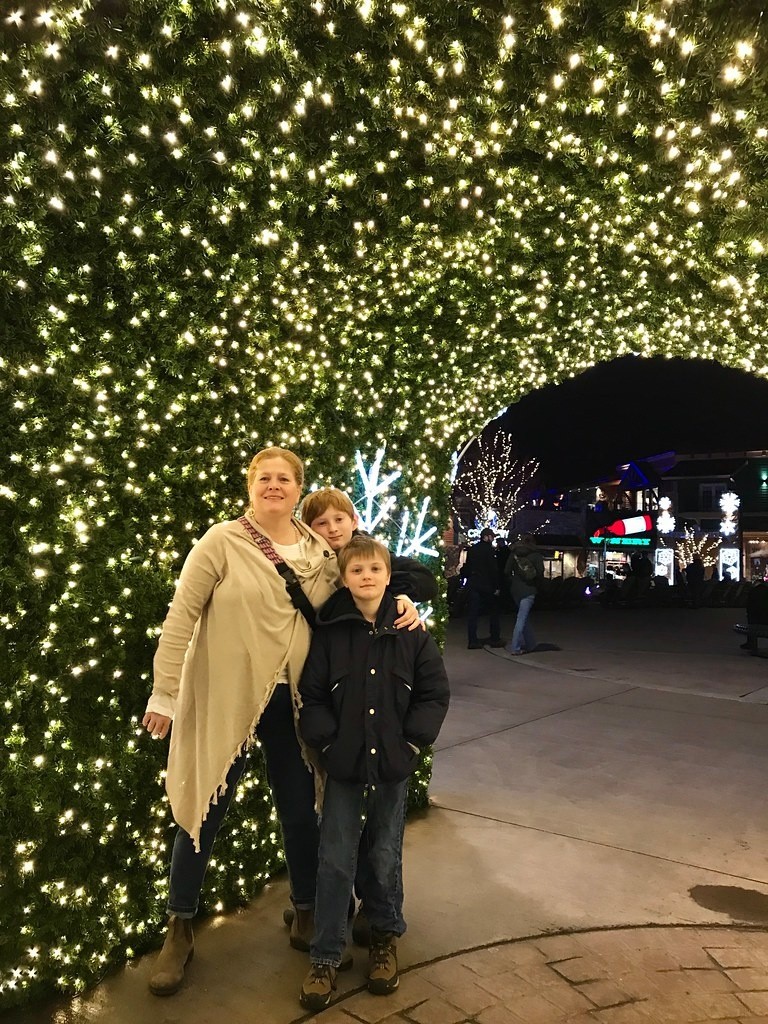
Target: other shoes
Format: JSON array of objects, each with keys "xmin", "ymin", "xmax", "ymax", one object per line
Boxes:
[
  {"xmin": 283, "ymin": 893, "xmax": 355, "ymax": 926},
  {"xmin": 512, "ymin": 649, "xmax": 528, "ymax": 656},
  {"xmin": 149, "ymin": 914, "xmax": 195, "ymax": 997},
  {"xmin": 352, "ymin": 898, "xmax": 371, "ymax": 946},
  {"xmin": 491, "ymin": 639, "xmax": 507, "ymax": 648},
  {"xmin": 468, "ymin": 639, "xmax": 483, "ymax": 649},
  {"xmin": 289, "ymin": 906, "xmax": 353, "ymax": 973},
  {"xmin": 528, "ymin": 644, "xmax": 537, "ymax": 652}
]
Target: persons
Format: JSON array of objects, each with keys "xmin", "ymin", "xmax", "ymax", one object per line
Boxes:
[
  {"xmin": 599, "ymin": 544, "xmax": 733, "ymax": 611},
  {"xmin": 459, "ymin": 529, "xmax": 508, "ymax": 649},
  {"xmin": 283, "ymin": 488, "xmax": 438, "ymax": 925},
  {"xmin": 504, "ymin": 533, "xmax": 545, "ymax": 655},
  {"xmin": 294, "ymin": 535, "xmax": 452, "ymax": 1011},
  {"xmin": 142, "ymin": 447, "xmax": 427, "ymax": 998},
  {"xmin": 739, "ymin": 578, "xmax": 768, "ymax": 651}
]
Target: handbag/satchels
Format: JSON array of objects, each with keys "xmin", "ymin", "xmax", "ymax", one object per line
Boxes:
[{"xmin": 512, "ymin": 553, "xmax": 538, "ymax": 582}]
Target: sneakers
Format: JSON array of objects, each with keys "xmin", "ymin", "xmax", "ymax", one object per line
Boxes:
[
  {"xmin": 300, "ymin": 964, "xmax": 338, "ymax": 1010},
  {"xmin": 368, "ymin": 932, "xmax": 400, "ymax": 996}
]
